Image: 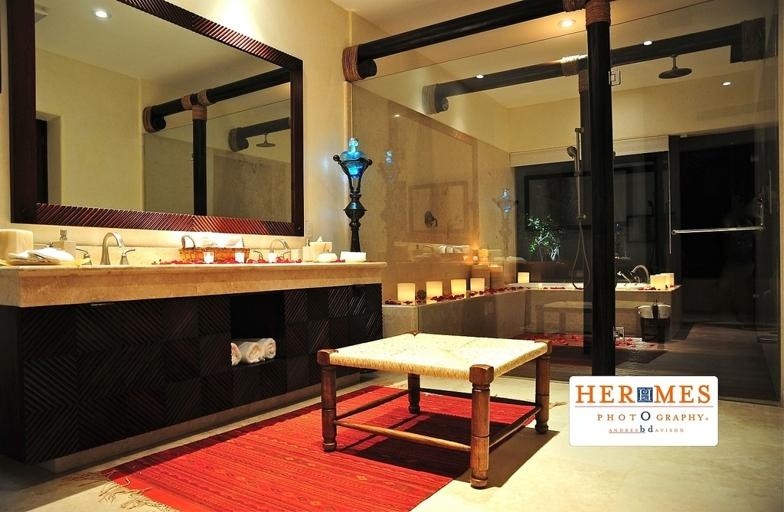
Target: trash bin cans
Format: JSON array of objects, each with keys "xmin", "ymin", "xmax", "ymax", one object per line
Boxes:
[{"xmin": 640, "ymin": 317, "xmax": 669, "ymax": 345}]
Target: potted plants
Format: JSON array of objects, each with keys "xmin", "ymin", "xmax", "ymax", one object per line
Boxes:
[{"xmin": 524, "ymin": 212, "xmax": 566, "ymax": 282}]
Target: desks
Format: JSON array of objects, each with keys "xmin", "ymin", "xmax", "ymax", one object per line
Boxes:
[
  {"xmin": 309, "ymin": 327, "xmax": 555, "ymax": 490},
  {"xmin": 534, "ymin": 297, "xmax": 663, "ymax": 347}
]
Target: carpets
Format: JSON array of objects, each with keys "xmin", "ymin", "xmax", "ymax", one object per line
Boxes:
[
  {"xmin": 514, "ymin": 325, "xmax": 640, "ymax": 371},
  {"xmin": 500, "ymin": 319, "xmax": 779, "ymax": 407},
  {"xmin": 66, "ymin": 381, "xmax": 574, "ymax": 512}
]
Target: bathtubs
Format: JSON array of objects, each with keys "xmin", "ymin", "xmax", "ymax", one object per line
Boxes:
[{"xmin": 507, "ymin": 282, "xmax": 684, "ymax": 339}]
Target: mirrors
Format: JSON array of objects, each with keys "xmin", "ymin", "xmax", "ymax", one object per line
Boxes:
[
  {"xmin": 350, "ymin": 85, "xmax": 482, "ymax": 248},
  {"xmin": 6, "ymin": 0, "xmax": 304, "ymax": 235}
]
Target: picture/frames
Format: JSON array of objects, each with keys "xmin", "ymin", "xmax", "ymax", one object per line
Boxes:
[{"xmin": 406, "ymin": 181, "xmax": 467, "ymax": 235}]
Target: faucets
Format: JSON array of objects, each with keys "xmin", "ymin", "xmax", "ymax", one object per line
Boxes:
[
  {"xmin": 269, "ymin": 239, "xmax": 290, "ymax": 253},
  {"xmin": 99, "ymin": 232, "xmax": 126, "ymax": 265},
  {"xmin": 629, "ymin": 265, "xmax": 650, "ymax": 281},
  {"xmin": 617, "ymin": 271, "xmax": 630, "ymax": 282}
]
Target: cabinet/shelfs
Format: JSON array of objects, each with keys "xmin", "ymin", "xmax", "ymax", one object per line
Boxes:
[{"xmin": 1, "ymin": 286, "xmax": 379, "ymax": 462}]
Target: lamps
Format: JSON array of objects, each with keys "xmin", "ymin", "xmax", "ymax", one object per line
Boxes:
[
  {"xmin": 664, "ymin": 274, "xmax": 677, "ymax": 288},
  {"xmin": 395, "ymin": 268, "xmax": 531, "ymax": 304},
  {"xmin": 649, "ymin": 272, "xmax": 667, "ymax": 291},
  {"xmin": 485, "ymin": 188, "xmax": 519, "ymax": 265},
  {"xmin": 331, "ymin": 139, "xmax": 372, "ymax": 252},
  {"xmin": 658, "ymin": 55, "xmax": 693, "ymax": 80},
  {"xmin": 254, "ymin": 132, "xmax": 276, "ymax": 149}
]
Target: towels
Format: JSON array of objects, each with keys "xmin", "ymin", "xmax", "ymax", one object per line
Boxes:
[{"xmin": 7, "ymin": 247, "xmax": 69, "ymax": 262}]
